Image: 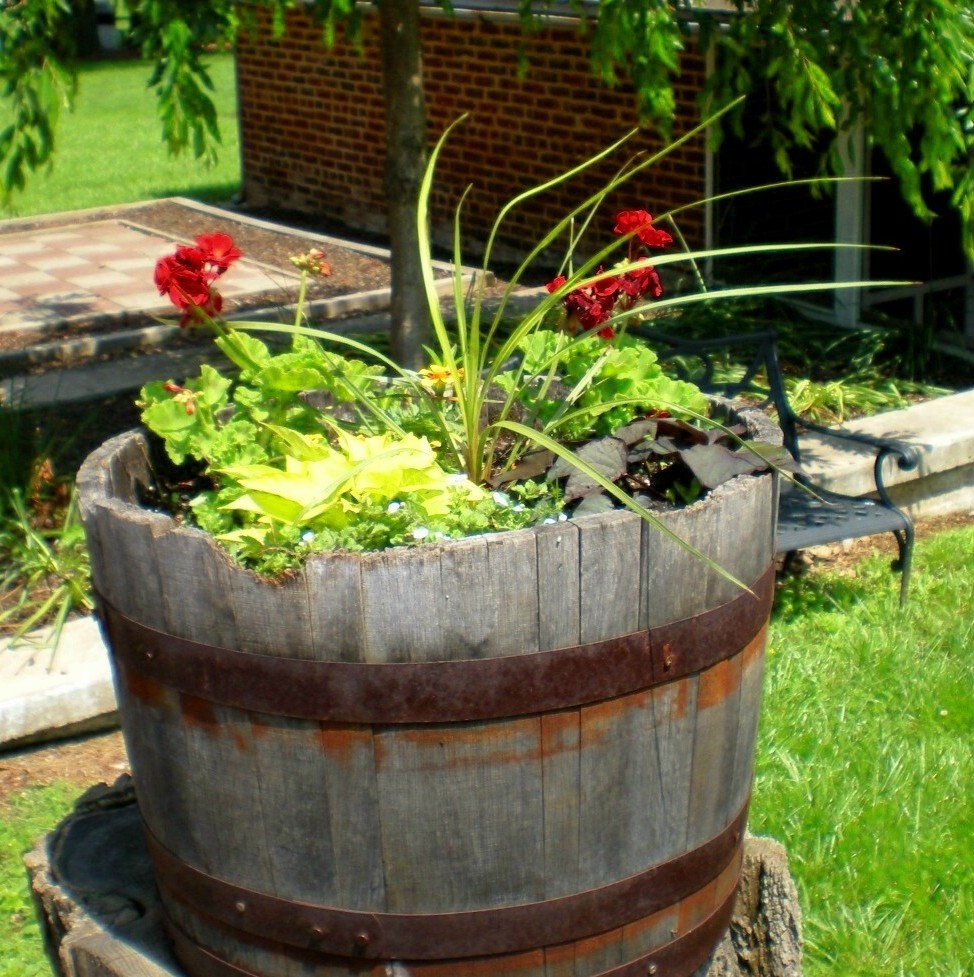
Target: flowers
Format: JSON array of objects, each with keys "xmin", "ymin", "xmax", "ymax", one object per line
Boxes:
[{"xmin": 134, "ymin": 92, "xmax": 922, "ymax": 602}]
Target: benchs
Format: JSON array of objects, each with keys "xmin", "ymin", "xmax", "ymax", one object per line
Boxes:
[{"xmin": 480, "ymin": 323, "xmax": 922, "ymax": 609}]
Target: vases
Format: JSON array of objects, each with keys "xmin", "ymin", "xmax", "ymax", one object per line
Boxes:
[{"xmin": 77, "ymin": 373, "xmax": 781, "ymax": 977}]
